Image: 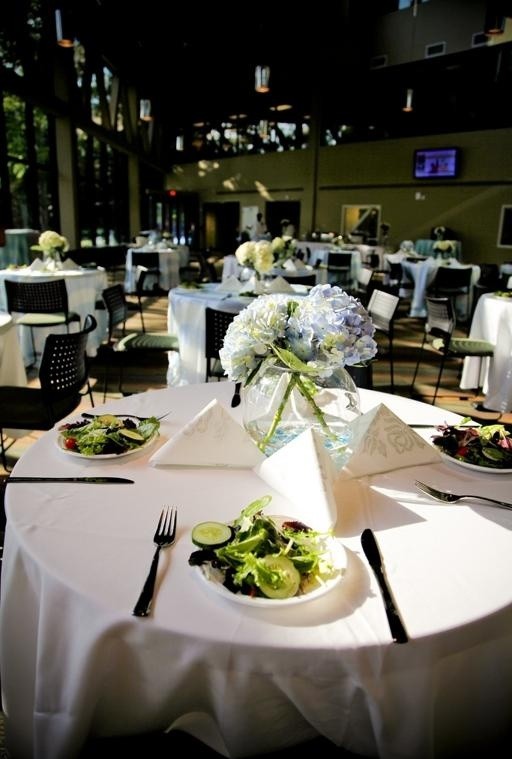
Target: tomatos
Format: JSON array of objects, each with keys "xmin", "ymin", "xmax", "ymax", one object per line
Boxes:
[
  {"xmin": 65, "ymin": 438, "xmax": 76, "ymax": 449},
  {"xmin": 457, "ymin": 447, "xmax": 468, "ymax": 457}
]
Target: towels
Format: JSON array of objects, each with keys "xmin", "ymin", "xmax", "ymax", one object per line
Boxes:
[
  {"xmin": 337, "ymin": 402, "xmax": 443, "ymax": 477},
  {"xmin": 253, "ymin": 426, "xmax": 339, "ymax": 528},
  {"xmin": 151, "ymin": 399, "xmax": 266, "ymax": 465}
]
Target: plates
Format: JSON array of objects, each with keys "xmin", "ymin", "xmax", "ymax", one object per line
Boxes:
[
  {"xmin": 432, "ymin": 436, "xmax": 512, "ymax": 475},
  {"xmin": 192, "ymin": 513, "xmax": 348, "ymax": 610},
  {"xmin": 56, "ymin": 420, "xmax": 159, "ymax": 461}
]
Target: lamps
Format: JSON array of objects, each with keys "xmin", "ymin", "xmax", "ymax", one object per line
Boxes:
[
  {"xmin": 176, "ymin": 134, "xmax": 184, "ymax": 151},
  {"xmin": 255, "ymin": 65, "xmax": 269, "ymax": 93},
  {"xmin": 404, "ymin": 87, "xmax": 414, "ymax": 112},
  {"xmin": 55, "ymin": 5, "xmax": 79, "ymax": 47},
  {"xmin": 138, "ymin": 98, "xmax": 152, "ymax": 121}
]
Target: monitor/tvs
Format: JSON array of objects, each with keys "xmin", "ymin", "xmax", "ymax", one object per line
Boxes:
[{"xmin": 412, "ymin": 147, "xmax": 458, "ymax": 179}]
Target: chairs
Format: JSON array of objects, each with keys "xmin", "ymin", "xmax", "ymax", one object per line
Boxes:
[
  {"xmin": 412, "ymin": 296, "xmax": 494, "ymax": 404},
  {"xmin": 362, "ymin": 290, "xmax": 401, "ymax": 384},
  {"xmin": 203, "ymin": 307, "xmax": 374, "ymax": 394},
  {"xmin": 1, "ymin": 231, "xmax": 192, "ymax": 473},
  {"xmin": 201, "ymin": 235, "xmax": 511, "ymax": 328}
]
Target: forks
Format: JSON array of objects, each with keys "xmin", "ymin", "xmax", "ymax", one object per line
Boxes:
[
  {"xmin": 131, "ymin": 503, "xmax": 179, "ymax": 620},
  {"xmin": 411, "ymin": 478, "xmax": 512, "ymax": 512},
  {"xmin": 81, "ymin": 411, "xmax": 174, "ymax": 424}
]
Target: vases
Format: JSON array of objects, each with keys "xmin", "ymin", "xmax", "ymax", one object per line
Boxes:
[{"xmin": 241, "ymin": 367, "xmax": 362, "ymax": 455}]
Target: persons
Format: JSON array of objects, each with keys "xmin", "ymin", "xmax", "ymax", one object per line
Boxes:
[
  {"xmin": 251, "ymin": 212, "xmax": 268, "ymax": 236},
  {"xmin": 280, "ymin": 218, "xmax": 295, "ymax": 239}
]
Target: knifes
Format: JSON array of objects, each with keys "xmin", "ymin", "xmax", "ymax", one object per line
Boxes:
[
  {"xmin": 360, "ymin": 529, "xmax": 406, "ymax": 646},
  {"xmin": 407, "ymin": 425, "xmax": 482, "ymax": 429},
  {"xmin": 2, "ymin": 474, "xmax": 138, "ymax": 485},
  {"xmin": 229, "ymin": 380, "xmax": 243, "ymax": 407}
]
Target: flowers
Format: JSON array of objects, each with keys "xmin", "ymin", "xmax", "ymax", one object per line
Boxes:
[{"xmin": 221, "ymin": 281, "xmax": 379, "ymax": 446}]
